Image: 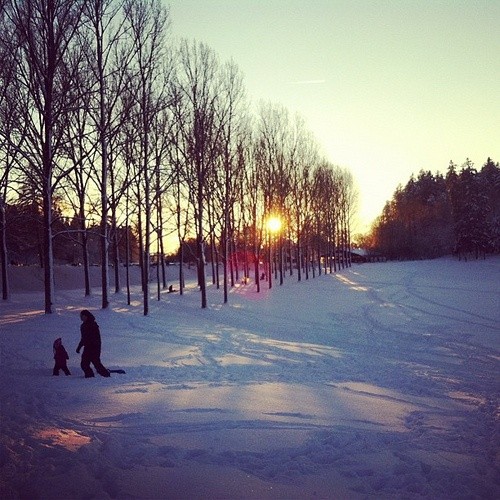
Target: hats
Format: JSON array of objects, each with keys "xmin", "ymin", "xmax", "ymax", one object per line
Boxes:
[{"xmin": 53, "ymin": 338, "xmax": 61, "ymax": 345}]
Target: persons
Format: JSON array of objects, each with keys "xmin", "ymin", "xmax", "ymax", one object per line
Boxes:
[
  {"xmin": 53, "ymin": 337, "xmax": 71, "ymax": 376},
  {"xmin": 76, "ymin": 310, "xmax": 111, "ymax": 378}
]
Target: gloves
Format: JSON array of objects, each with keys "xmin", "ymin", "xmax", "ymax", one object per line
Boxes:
[{"xmin": 76, "ymin": 347, "xmax": 80, "ymax": 354}]
